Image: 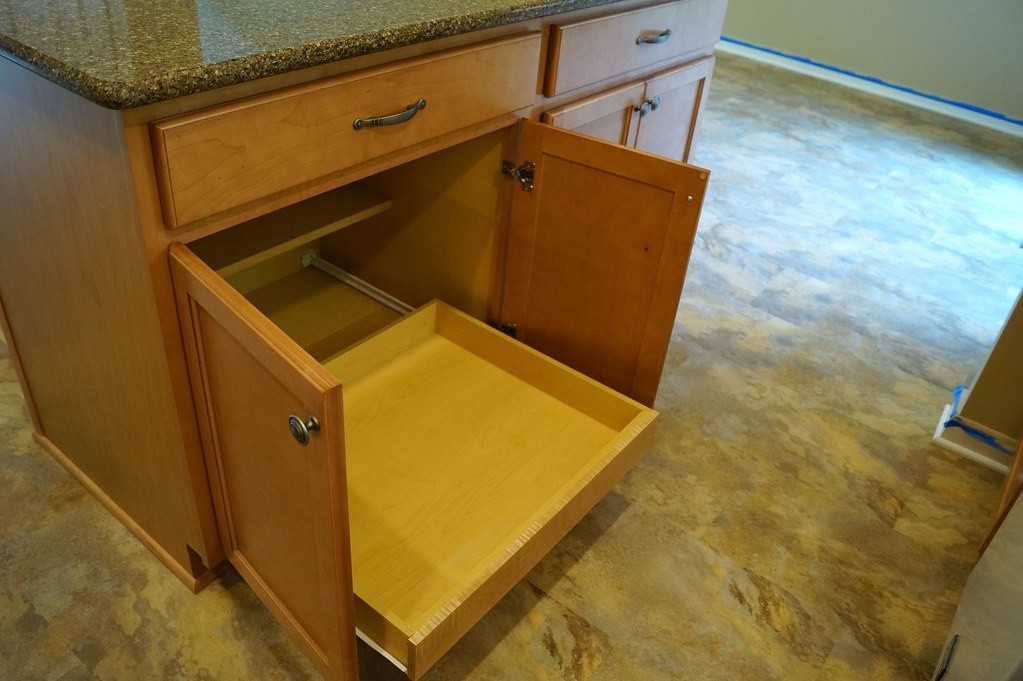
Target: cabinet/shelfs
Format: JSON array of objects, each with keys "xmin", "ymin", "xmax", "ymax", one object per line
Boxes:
[{"xmin": 1, "ymin": 1, "xmax": 725, "ymax": 680}]
